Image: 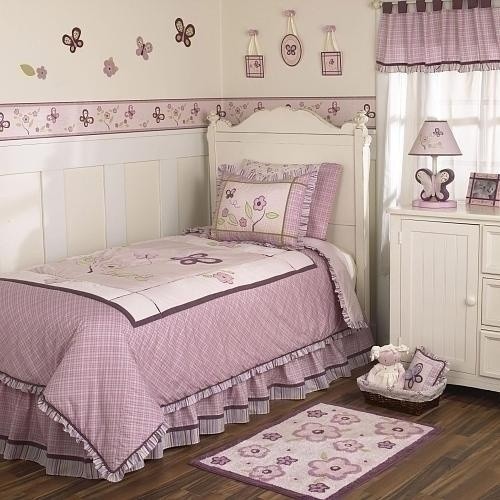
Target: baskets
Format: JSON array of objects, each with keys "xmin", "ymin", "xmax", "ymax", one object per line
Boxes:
[{"xmin": 356, "ymin": 362, "xmax": 448, "ymax": 416}]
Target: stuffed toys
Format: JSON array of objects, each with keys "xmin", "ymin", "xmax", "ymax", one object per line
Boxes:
[{"xmin": 366, "ymin": 344, "xmax": 409, "ymax": 390}]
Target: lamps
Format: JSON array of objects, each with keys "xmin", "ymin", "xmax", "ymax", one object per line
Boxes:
[{"xmin": 407, "ymin": 120, "xmax": 463, "ymax": 209}]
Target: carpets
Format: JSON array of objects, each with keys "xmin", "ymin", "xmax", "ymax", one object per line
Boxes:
[{"xmin": 187, "ymin": 401, "xmax": 446, "ymax": 500}]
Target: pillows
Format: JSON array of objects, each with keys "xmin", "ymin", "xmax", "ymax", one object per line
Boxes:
[{"xmin": 210, "ymin": 158, "xmax": 344, "ymax": 251}]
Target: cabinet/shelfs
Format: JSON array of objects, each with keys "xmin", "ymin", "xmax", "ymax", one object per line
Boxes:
[{"xmin": 387, "ymin": 205, "xmax": 500, "ymax": 393}]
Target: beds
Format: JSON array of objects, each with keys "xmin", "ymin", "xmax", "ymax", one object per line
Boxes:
[{"xmin": 1, "ymin": 106, "xmax": 373, "ymax": 483}]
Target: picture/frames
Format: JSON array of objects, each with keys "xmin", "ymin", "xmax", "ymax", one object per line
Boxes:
[{"xmin": 465, "ymin": 172, "xmax": 500, "ymax": 207}]
